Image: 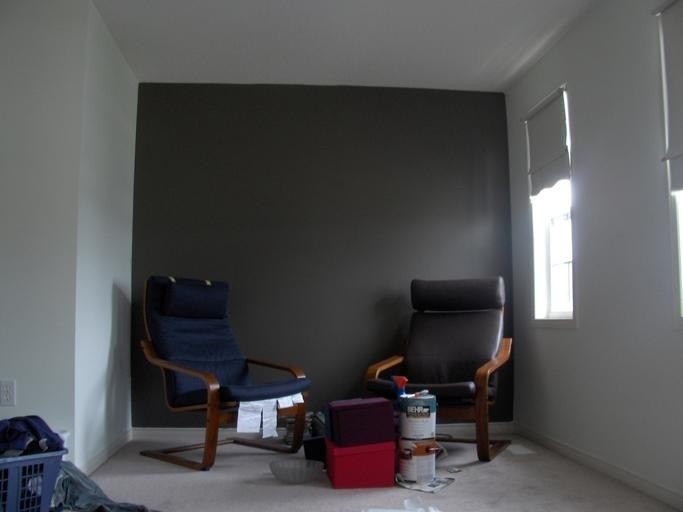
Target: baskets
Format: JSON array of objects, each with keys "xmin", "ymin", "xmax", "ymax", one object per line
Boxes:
[{"xmin": 0, "ymin": 450, "xmax": 68, "ymax": 512}]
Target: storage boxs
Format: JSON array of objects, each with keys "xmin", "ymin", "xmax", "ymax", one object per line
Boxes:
[{"xmin": 323, "ymin": 436, "xmax": 395, "ymax": 488}]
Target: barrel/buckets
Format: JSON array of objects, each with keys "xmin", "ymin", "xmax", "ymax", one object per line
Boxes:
[
  {"xmin": 397, "ymin": 438, "xmax": 440, "ymax": 481},
  {"xmin": 401, "ymin": 392, "xmax": 438, "ymax": 439}
]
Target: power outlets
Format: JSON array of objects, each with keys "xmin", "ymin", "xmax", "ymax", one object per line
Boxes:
[{"xmin": 0, "ymin": 381, "xmax": 13, "ymax": 405}]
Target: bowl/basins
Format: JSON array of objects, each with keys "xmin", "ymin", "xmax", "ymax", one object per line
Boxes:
[{"xmin": 270, "ymin": 458, "xmax": 326, "ymax": 484}]
